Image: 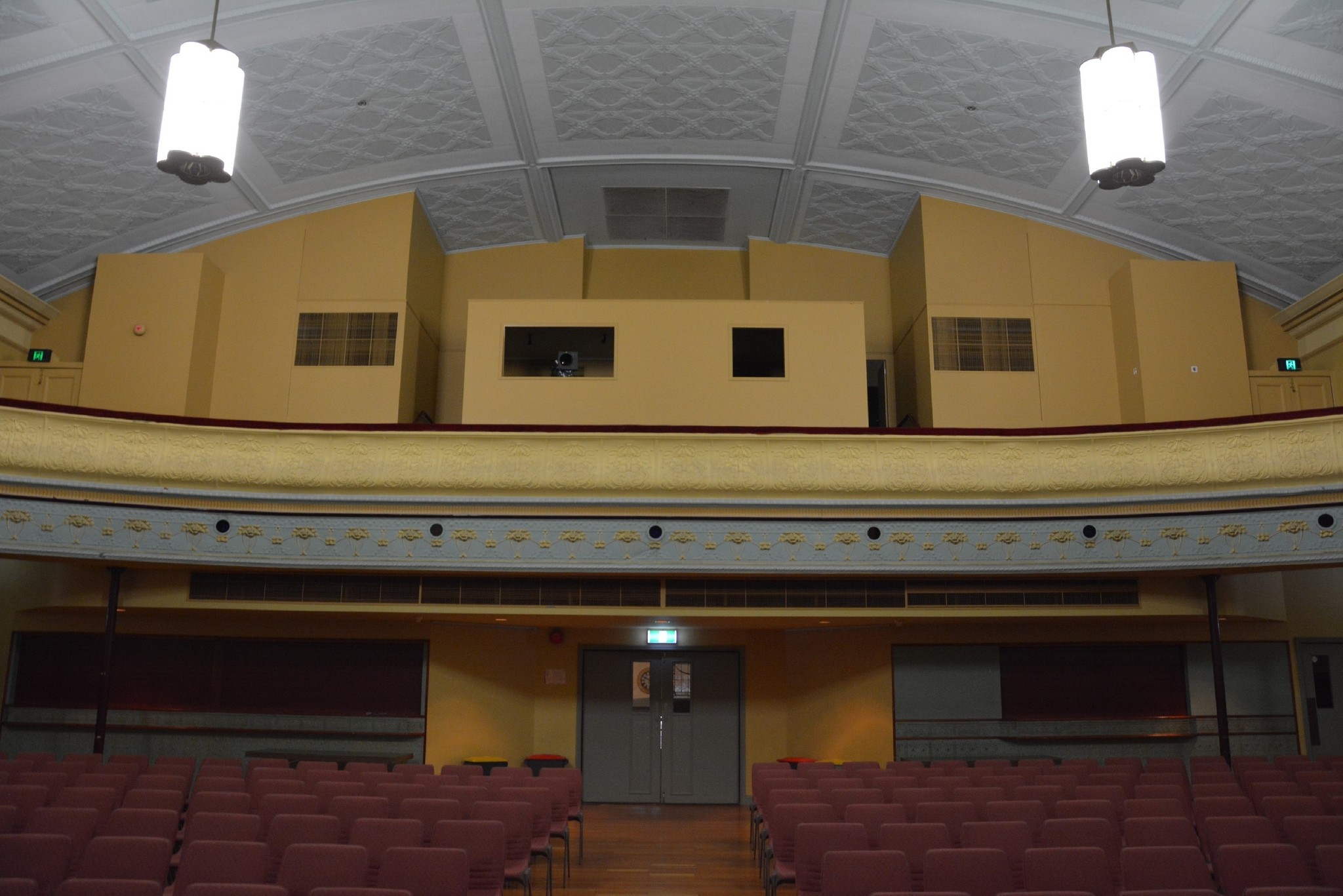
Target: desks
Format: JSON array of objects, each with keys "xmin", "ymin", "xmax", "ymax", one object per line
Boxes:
[
  {"xmin": 244, "ymin": 746, "xmax": 413, "ymax": 771},
  {"xmin": 900, "ymin": 755, "xmax": 1063, "ymax": 769}
]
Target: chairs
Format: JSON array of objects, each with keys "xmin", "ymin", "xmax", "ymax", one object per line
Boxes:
[
  {"xmin": 747, "ymin": 756, "xmax": 1343, "ymax": 896},
  {"xmin": 0, "ymin": 752, "xmax": 585, "ymax": 896}
]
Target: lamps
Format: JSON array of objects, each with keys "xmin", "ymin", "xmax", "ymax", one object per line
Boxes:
[
  {"xmin": 1078, "ymin": 0, "xmax": 1166, "ymax": 190},
  {"xmin": 153, "ymin": 1, "xmax": 246, "ymax": 186}
]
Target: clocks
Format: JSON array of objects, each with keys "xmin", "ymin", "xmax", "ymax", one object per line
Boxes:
[{"xmin": 637, "ymin": 667, "xmax": 650, "ymax": 694}]
